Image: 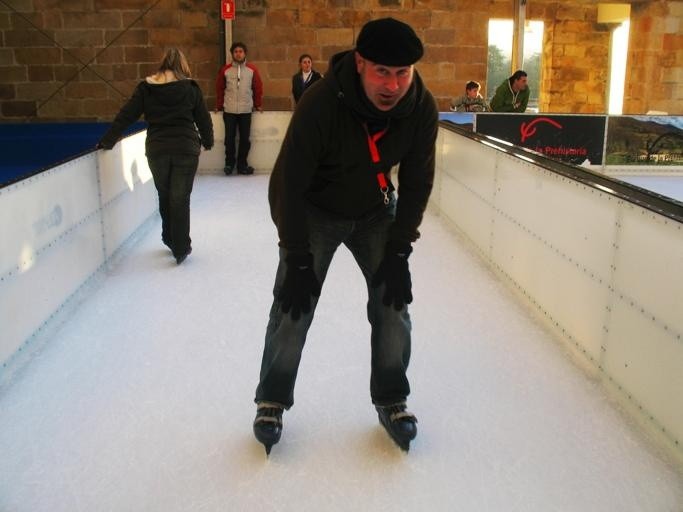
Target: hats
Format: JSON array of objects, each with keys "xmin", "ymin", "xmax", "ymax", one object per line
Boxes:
[{"xmin": 354, "ymin": 17, "xmax": 425, "ymax": 68}]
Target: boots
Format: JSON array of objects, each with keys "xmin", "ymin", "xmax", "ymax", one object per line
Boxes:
[{"xmin": 224, "ymin": 162, "xmax": 254, "ymax": 175}]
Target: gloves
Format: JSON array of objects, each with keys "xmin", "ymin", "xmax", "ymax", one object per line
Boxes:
[
  {"xmin": 272, "ymin": 237, "xmax": 321, "ymax": 322},
  {"xmin": 369, "ymin": 235, "xmax": 413, "ymax": 312}
]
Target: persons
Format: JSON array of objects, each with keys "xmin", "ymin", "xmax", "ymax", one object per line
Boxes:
[
  {"xmin": 253, "ymin": 17, "xmax": 439, "ymax": 455},
  {"xmin": 490, "ymin": 70, "xmax": 530, "ymax": 113},
  {"xmin": 292, "ymin": 54, "xmax": 322, "ymax": 105},
  {"xmin": 214, "ymin": 41, "xmax": 265, "ymax": 175},
  {"xmin": 95, "ymin": 47, "xmax": 214, "ymax": 265},
  {"xmin": 449, "ymin": 80, "xmax": 493, "ymax": 112}
]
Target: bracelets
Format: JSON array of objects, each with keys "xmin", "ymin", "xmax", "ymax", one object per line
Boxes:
[{"xmin": 466, "ymin": 105, "xmax": 469, "ymax": 112}]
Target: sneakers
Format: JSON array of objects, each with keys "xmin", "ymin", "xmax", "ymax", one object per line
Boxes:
[
  {"xmin": 252, "ymin": 401, "xmax": 284, "ymax": 445},
  {"xmin": 375, "ymin": 399, "xmax": 418, "ymax": 442}
]
